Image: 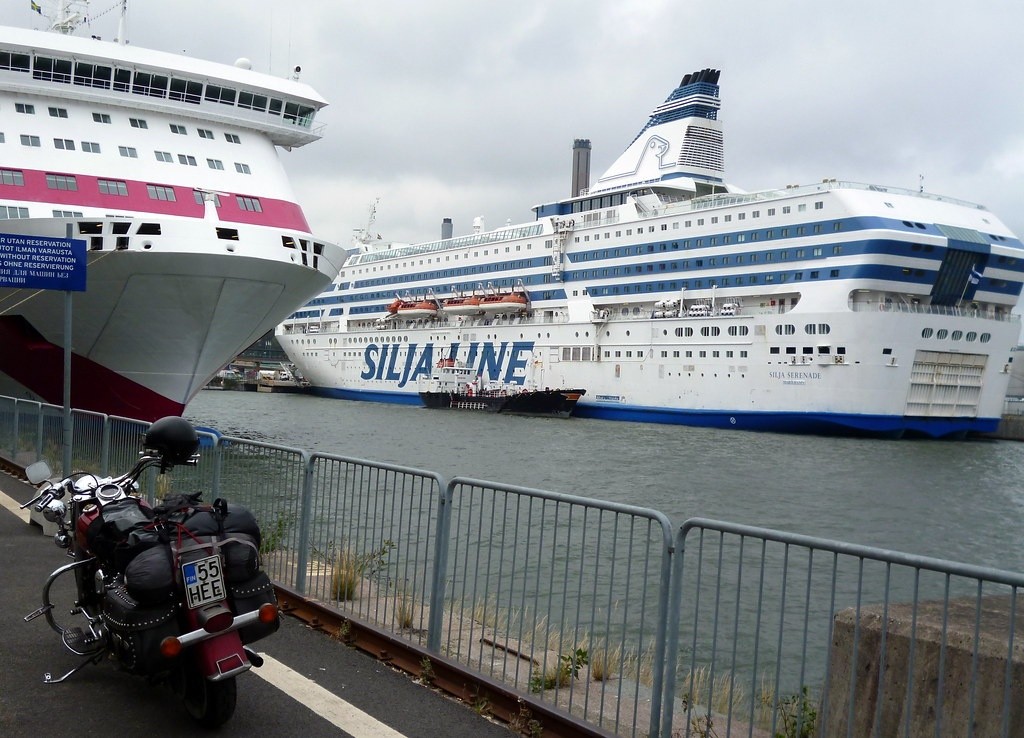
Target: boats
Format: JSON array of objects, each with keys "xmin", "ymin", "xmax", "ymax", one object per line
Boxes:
[
  {"xmin": 386, "ymin": 300, "xmax": 404, "ymax": 313},
  {"xmin": 398, "ymin": 303, "xmax": 438, "ymax": 320},
  {"xmin": 415, "ymin": 347, "xmax": 588, "ymax": 419},
  {"xmin": 442, "ymin": 296, "xmax": 481, "ymax": 316},
  {"xmin": 478, "ymin": 294, "xmax": 528, "ymax": 315}
]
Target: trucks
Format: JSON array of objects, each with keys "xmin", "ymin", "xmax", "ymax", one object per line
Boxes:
[
  {"xmin": 279, "ymin": 372, "xmax": 290, "ymax": 381},
  {"xmin": 213, "ymin": 370, "xmax": 244, "ymax": 380}
]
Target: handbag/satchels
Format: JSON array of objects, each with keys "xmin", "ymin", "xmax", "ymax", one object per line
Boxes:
[
  {"xmin": 99, "ymin": 585, "xmax": 182, "ymax": 681},
  {"xmin": 223, "ymin": 567, "xmax": 280, "ymax": 646}
]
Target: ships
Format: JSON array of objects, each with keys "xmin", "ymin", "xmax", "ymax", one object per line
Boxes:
[
  {"xmin": 274, "ymin": 68, "xmax": 1024, "ymax": 441},
  {"xmin": 0, "ymin": 0, "xmax": 351, "ymax": 444}
]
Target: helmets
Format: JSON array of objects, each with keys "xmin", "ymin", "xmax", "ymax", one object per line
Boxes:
[{"xmin": 145, "ymin": 415, "xmax": 201, "ymax": 466}]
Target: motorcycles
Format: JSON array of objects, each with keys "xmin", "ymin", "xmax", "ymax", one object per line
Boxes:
[{"xmin": 18, "ymin": 459, "xmax": 280, "ymax": 732}]
